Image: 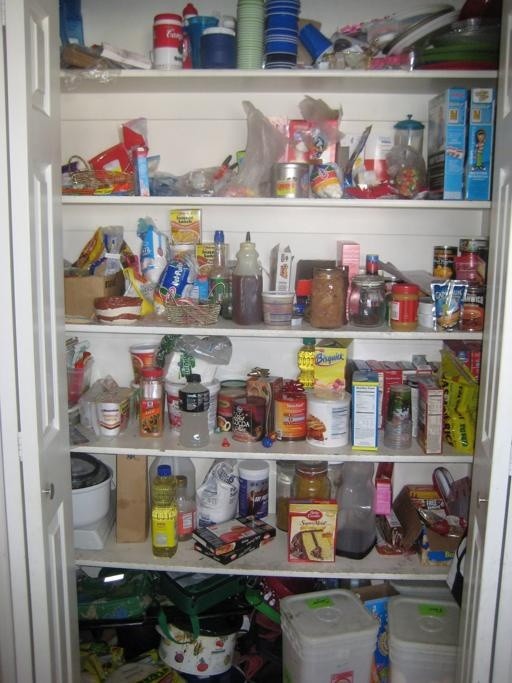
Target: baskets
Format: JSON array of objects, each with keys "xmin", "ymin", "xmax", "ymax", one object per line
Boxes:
[{"xmin": 158, "ymin": 283, "xmax": 225, "ymax": 327}]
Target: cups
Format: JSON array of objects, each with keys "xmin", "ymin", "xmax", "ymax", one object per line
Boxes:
[
  {"xmin": 382, "ymin": 383, "xmax": 413, "ymax": 450},
  {"xmin": 184, "ymin": 0, "xmax": 335, "ymax": 71},
  {"xmin": 151, "ymin": 13, "xmax": 183, "ymax": 71},
  {"xmin": 141, "ymin": 232, "xmax": 196, "ymax": 319}
]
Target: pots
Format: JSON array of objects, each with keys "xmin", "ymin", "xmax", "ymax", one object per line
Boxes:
[{"xmin": 70, "ymin": 452, "xmax": 113, "ymax": 529}]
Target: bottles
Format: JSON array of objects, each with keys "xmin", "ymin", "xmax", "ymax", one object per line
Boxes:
[
  {"xmin": 289, "ymin": 460, "xmax": 331, "ymax": 561},
  {"xmin": 276, "ymin": 460, "xmax": 295, "ymax": 532},
  {"xmin": 296, "ymin": 337, "xmax": 316, "ymax": 389},
  {"xmin": 139, "ymin": 367, "xmax": 165, "ymax": 439},
  {"xmin": 150, "ymin": 464, "xmax": 178, "ymax": 559},
  {"xmin": 176, "ymin": 475, "xmax": 197, "ymax": 542},
  {"xmin": 178, "ymin": 373, "xmax": 210, "ymax": 449},
  {"xmin": 238, "ymin": 459, "xmax": 270, "ymax": 520},
  {"xmin": 392, "ymin": 113, "xmax": 424, "ymax": 155},
  {"xmin": 208, "ymin": 231, "xmax": 490, "ymax": 333}
]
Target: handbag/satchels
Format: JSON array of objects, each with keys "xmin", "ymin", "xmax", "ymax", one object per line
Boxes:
[
  {"xmin": 156, "ymin": 573, "xmax": 280, "ymax": 645},
  {"xmin": 77, "ymin": 568, "xmax": 151, "ymax": 622}
]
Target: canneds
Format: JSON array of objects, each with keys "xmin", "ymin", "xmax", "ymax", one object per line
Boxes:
[
  {"xmin": 270, "ymin": 162, "xmax": 309, "ymax": 198},
  {"xmin": 276, "ymin": 460, "xmax": 342, "ymax": 532},
  {"xmin": 218, "ymin": 379, "xmax": 267, "ymax": 444},
  {"xmin": 432, "ymin": 245, "xmax": 458, "ymax": 281},
  {"xmin": 458, "ymin": 286, "xmax": 486, "ymax": 332}
]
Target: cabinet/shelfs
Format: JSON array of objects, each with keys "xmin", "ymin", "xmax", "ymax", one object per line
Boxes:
[{"xmin": 0, "ymin": 0, "xmax": 512, "ymax": 680}]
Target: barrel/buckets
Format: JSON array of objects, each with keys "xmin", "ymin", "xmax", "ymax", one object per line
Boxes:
[
  {"xmin": 148, "ymin": 456, "xmax": 196, "ymax": 505},
  {"xmin": 164, "ymin": 379, "xmax": 222, "ymax": 437},
  {"xmin": 279, "ymin": 588, "xmax": 380, "ymax": 682},
  {"xmin": 334, "ymin": 462, "xmax": 378, "ymax": 561},
  {"xmin": 387, "ymin": 595, "xmax": 459, "ymax": 682}
]
